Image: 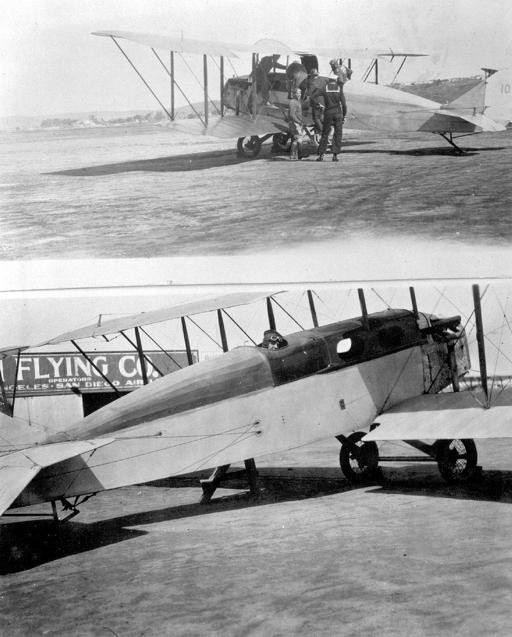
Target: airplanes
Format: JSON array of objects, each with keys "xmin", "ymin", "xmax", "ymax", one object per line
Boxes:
[
  {"xmin": 84, "ymin": 26, "xmax": 510, "ymax": 159},
  {"xmin": 0, "ymin": 289, "xmax": 512, "ymax": 539}
]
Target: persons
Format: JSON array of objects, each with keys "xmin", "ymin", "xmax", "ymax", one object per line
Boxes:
[
  {"xmin": 329, "ymin": 58, "xmax": 353, "ymax": 85},
  {"xmin": 308, "ymin": 75, "xmax": 347, "ymax": 162},
  {"xmin": 252, "ymin": 52, "xmax": 332, "ymax": 137},
  {"xmin": 285, "ymin": 86, "xmax": 307, "ymax": 160},
  {"xmin": 262, "ymin": 329, "xmax": 284, "ymax": 351}
]
